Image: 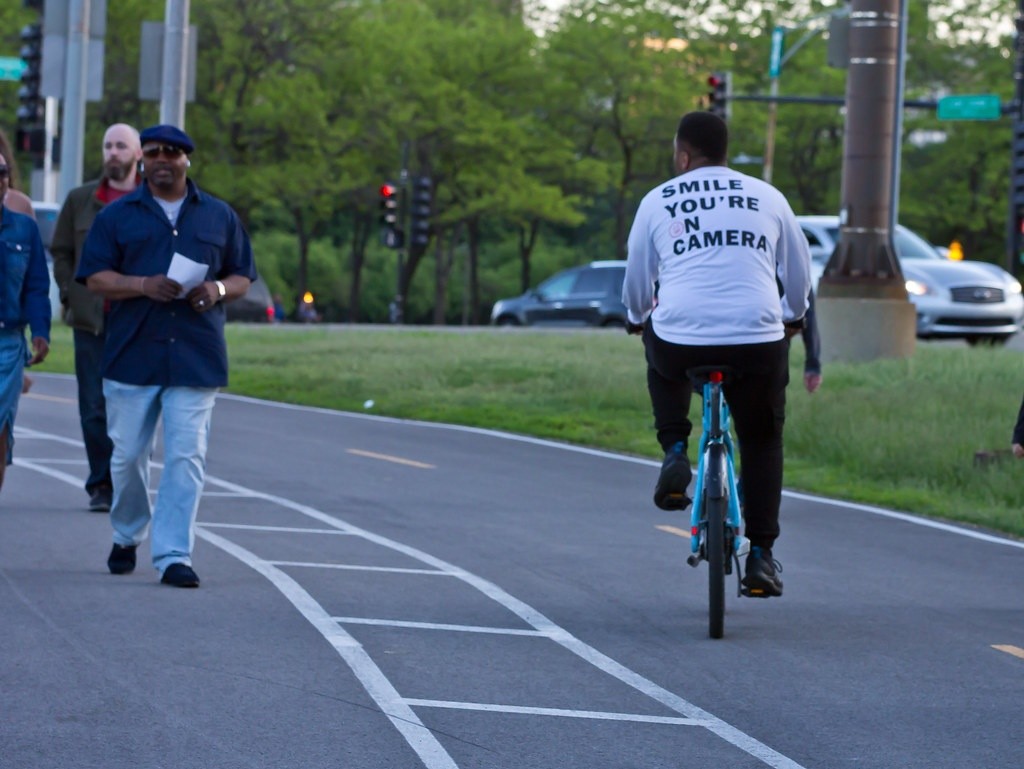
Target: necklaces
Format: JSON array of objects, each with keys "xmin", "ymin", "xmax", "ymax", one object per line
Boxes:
[{"xmin": 163, "ymin": 205, "xmax": 179, "ymax": 221}]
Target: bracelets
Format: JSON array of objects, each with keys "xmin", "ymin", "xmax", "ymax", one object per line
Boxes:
[{"xmin": 215, "ymin": 280, "xmax": 226, "ymax": 301}]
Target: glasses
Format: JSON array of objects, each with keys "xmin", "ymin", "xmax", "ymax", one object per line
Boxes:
[
  {"xmin": 0, "ymin": 164, "xmax": 11, "ymax": 178},
  {"xmin": 143, "ymin": 144, "xmax": 185, "ymax": 159}
]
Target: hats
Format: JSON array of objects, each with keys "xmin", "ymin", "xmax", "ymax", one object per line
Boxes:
[{"xmin": 140, "ymin": 125, "xmax": 195, "ymax": 154}]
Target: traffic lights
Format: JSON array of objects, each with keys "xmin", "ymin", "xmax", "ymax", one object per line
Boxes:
[
  {"xmin": 706, "ymin": 70, "xmax": 731, "ymax": 116},
  {"xmin": 380, "ymin": 183, "xmax": 404, "ymax": 229},
  {"xmin": 13, "ymin": 24, "xmax": 43, "ymax": 124},
  {"xmin": 1011, "ymin": 119, "xmax": 1024, "ymax": 219},
  {"xmin": 16, "ymin": 128, "xmax": 43, "ymax": 150},
  {"xmin": 408, "ymin": 175, "xmax": 435, "ymax": 250}
]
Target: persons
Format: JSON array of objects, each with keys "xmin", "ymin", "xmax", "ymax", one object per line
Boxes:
[
  {"xmin": 1011, "ymin": 396, "xmax": 1024, "ymax": 458},
  {"xmin": 49, "ymin": 123, "xmax": 142, "ymax": 511},
  {"xmin": 75, "ymin": 125, "xmax": 257, "ymax": 588},
  {"xmin": 621, "ymin": 111, "xmax": 822, "ymax": 597},
  {"xmin": 0, "ymin": 130, "xmax": 52, "ymax": 489}
]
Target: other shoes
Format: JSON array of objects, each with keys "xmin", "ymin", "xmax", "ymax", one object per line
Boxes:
[
  {"xmin": 161, "ymin": 563, "xmax": 200, "ymax": 588},
  {"xmin": 89, "ymin": 485, "xmax": 112, "ymax": 511},
  {"xmin": 107, "ymin": 543, "xmax": 136, "ymax": 575}
]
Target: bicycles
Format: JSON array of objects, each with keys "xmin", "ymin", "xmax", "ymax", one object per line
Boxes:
[{"xmin": 663, "ymin": 363, "xmax": 773, "ymax": 640}]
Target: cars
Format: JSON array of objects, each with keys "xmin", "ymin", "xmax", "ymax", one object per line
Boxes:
[
  {"xmin": 793, "ymin": 215, "xmax": 1023, "ymax": 348},
  {"xmin": 224, "ymin": 273, "xmax": 273, "ymax": 323},
  {"xmin": 488, "ymin": 260, "xmax": 632, "ymax": 329}
]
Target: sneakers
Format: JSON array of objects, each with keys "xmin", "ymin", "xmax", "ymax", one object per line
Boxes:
[
  {"xmin": 741, "ymin": 544, "xmax": 784, "ymax": 596},
  {"xmin": 653, "ymin": 443, "xmax": 693, "ymax": 511}
]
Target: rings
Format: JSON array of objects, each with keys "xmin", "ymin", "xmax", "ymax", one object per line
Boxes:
[{"xmin": 199, "ymin": 300, "xmax": 205, "ymax": 307}]
